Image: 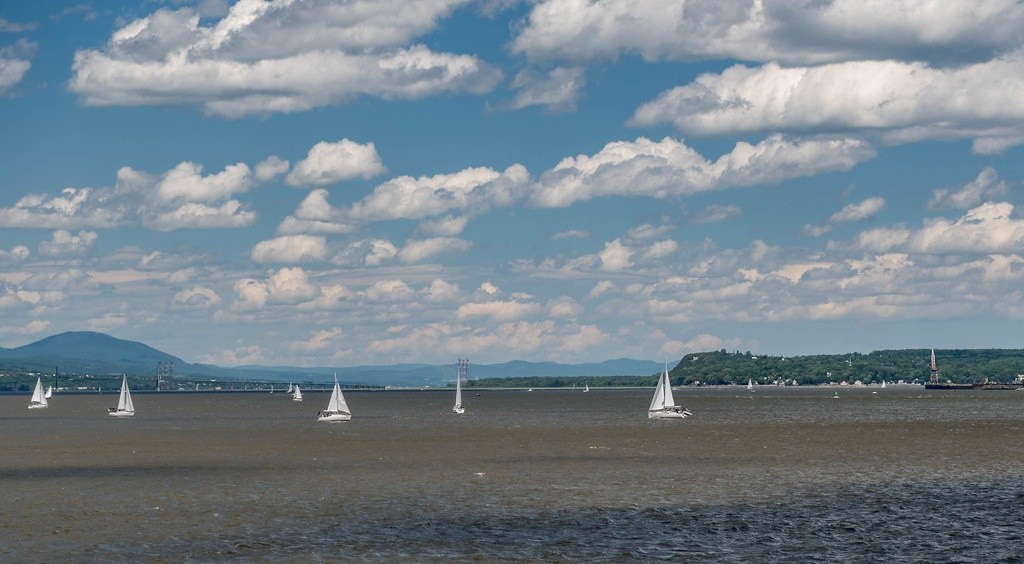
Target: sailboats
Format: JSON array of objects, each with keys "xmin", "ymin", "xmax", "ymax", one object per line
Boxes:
[
  {"xmin": 647, "ymin": 362, "xmax": 692, "ymax": 419},
  {"xmin": 108, "ymin": 374, "xmax": 135, "ymax": 417},
  {"xmin": 291, "ymin": 385, "xmax": 303, "ymax": 402},
  {"xmin": 286, "ymin": 382, "xmax": 294, "ymax": 393},
  {"xmin": 44, "ymin": 386, "xmax": 52, "ymax": 399},
  {"xmin": 746, "ymin": 379, "xmax": 753, "ymax": 390},
  {"xmin": 453, "ymin": 358, "xmax": 469, "ymax": 413},
  {"xmin": 583, "ymin": 383, "xmax": 590, "ymax": 393},
  {"xmin": 317, "ymin": 372, "xmax": 352, "ymax": 422},
  {"xmin": 27, "ymin": 377, "xmax": 49, "ymax": 409},
  {"xmin": 881, "ymin": 380, "xmax": 886, "ymax": 389}
]
